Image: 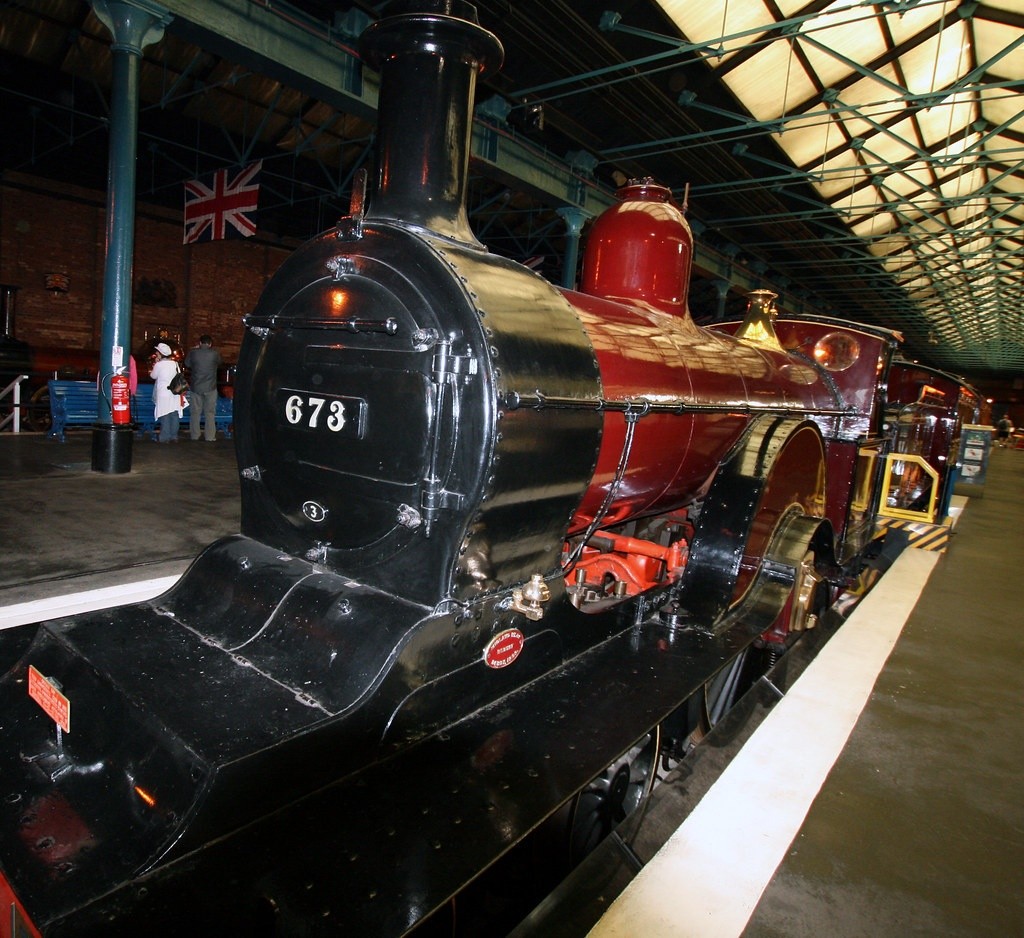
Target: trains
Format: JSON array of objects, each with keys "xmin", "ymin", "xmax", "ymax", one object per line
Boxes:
[{"xmin": 1, "ymin": 0, "xmax": 997, "ymax": 938}]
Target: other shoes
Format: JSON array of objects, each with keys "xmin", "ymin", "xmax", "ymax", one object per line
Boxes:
[{"xmin": 205, "ymin": 438, "xmax": 217, "ymax": 442}]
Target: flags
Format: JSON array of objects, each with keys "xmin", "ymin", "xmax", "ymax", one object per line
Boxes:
[{"xmin": 182, "ymin": 158, "xmax": 263, "ymax": 246}]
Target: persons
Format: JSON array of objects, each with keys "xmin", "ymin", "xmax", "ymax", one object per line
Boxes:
[
  {"xmin": 184, "ymin": 334, "xmax": 222, "ymax": 442},
  {"xmin": 150, "ymin": 342, "xmax": 190, "ymax": 445}
]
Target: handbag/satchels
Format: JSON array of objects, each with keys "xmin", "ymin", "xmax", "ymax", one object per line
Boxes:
[{"xmin": 169, "ymin": 373, "xmax": 189, "ymax": 395}]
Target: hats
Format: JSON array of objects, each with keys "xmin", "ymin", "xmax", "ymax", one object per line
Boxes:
[{"xmin": 156, "ymin": 343, "xmax": 171, "ymax": 357}]
